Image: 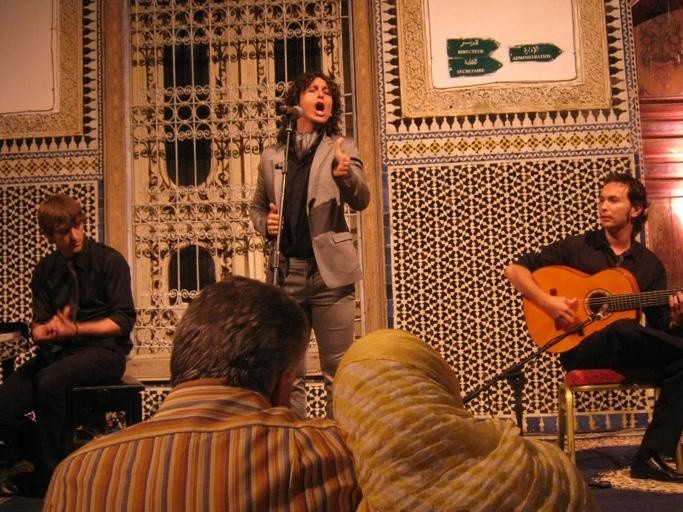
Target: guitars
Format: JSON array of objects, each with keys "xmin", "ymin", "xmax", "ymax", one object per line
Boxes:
[{"xmin": 522, "ymin": 265, "xmax": 683, "ymax": 353}]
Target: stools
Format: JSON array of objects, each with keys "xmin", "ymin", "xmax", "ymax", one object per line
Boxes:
[
  {"xmin": 557, "ymin": 368, "xmax": 683, "ymax": 475},
  {"xmin": 65, "ymin": 375, "xmax": 146, "ymax": 452}
]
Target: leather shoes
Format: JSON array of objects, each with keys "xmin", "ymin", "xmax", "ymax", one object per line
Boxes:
[{"xmin": 630, "ymin": 454, "xmax": 683, "ymax": 483}]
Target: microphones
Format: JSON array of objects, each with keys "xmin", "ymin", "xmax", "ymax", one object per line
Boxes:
[
  {"xmin": 277, "ymin": 104, "xmax": 303, "ymax": 119},
  {"xmin": 595, "ymin": 304, "xmax": 609, "ymax": 320}
]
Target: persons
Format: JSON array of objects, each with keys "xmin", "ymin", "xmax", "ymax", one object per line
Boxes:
[
  {"xmin": 248, "ymin": 72, "xmax": 370, "ymax": 421},
  {"xmin": 42, "ymin": 272, "xmax": 361, "ymax": 512},
  {"xmin": 503, "ymin": 171, "xmax": 679, "ymax": 481},
  {"xmin": 0, "ymin": 194, "xmax": 137, "ymax": 495},
  {"xmin": 332, "ymin": 329, "xmax": 598, "ymax": 511}
]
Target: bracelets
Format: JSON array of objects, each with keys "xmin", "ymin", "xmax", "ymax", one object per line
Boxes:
[{"xmin": 75, "ymin": 322, "xmax": 79, "ymax": 336}]
